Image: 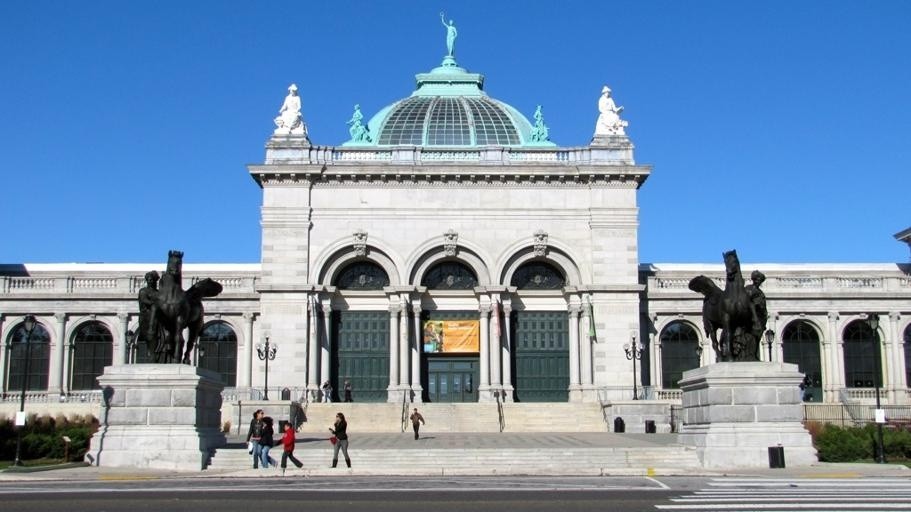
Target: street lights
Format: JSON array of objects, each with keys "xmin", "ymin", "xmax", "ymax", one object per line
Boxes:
[
  {"xmin": 15, "ymin": 314, "xmax": 36, "ymax": 465},
  {"xmin": 623, "ymin": 331, "xmax": 646, "ymax": 399},
  {"xmin": 256, "ymin": 334, "xmax": 276, "ymax": 400},
  {"xmin": 766, "ymin": 330, "xmax": 777, "ymax": 363},
  {"xmin": 866, "ymin": 312, "xmax": 887, "ymax": 464}
]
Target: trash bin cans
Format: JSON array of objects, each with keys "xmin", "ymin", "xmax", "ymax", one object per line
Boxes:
[
  {"xmin": 768, "ymin": 447, "xmax": 785, "ymax": 468},
  {"xmin": 282, "ymin": 388, "xmax": 290, "ymax": 400},
  {"xmin": 645, "ymin": 419, "xmax": 654, "ymax": 433},
  {"xmin": 279, "ymin": 420, "xmax": 288, "ymax": 433},
  {"xmin": 614, "ymin": 417, "xmax": 624, "ymax": 432}
]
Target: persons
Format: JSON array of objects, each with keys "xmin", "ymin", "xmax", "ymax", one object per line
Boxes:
[
  {"xmin": 247, "ymin": 408, "xmax": 275, "ymax": 468},
  {"xmin": 328, "ymin": 412, "xmax": 351, "ymax": 467},
  {"xmin": 258, "ymin": 416, "xmax": 279, "ymax": 468},
  {"xmin": 276, "ymin": 422, "xmax": 304, "ymax": 467},
  {"xmin": 343, "ymin": 376, "xmax": 354, "ymax": 402},
  {"xmin": 424, "ymin": 321, "xmax": 442, "ymax": 353},
  {"xmin": 746, "ymin": 269, "xmax": 769, "ymax": 362},
  {"xmin": 136, "ymin": 271, "xmax": 163, "ymax": 363},
  {"xmin": 410, "ymin": 408, "xmax": 425, "ymax": 439},
  {"xmin": 534, "ymin": 102, "xmax": 546, "ymax": 142},
  {"xmin": 279, "ymin": 83, "xmax": 303, "ymax": 137},
  {"xmin": 347, "ymin": 104, "xmax": 365, "ymax": 140},
  {"xmin": 323, "ymin": 379, "xmax": 335, "ymax": 402},
  {"xmin": 595, "ymin": 85, "xmax": 630, "ymax": 137},
  {"xmin": 441, "ymin": 11, "xmax": 459, "ymax": 55}
]
